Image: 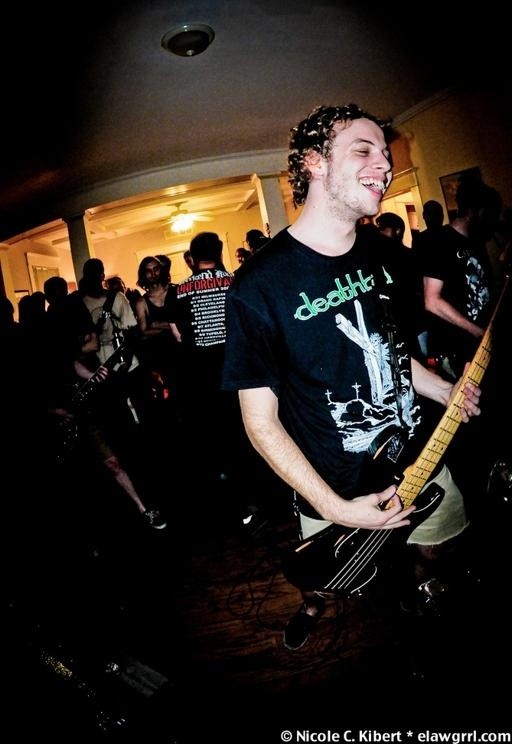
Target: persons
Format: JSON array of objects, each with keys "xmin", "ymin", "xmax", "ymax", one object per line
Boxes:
[{"xmin": 2, "ymin": 101, "xmax": 512, "ymax": 743}]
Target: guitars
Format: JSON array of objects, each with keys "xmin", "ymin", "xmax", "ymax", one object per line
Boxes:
[{"xmin": 284, "ymin": 273, "xmax": 505, "ymax": 599}]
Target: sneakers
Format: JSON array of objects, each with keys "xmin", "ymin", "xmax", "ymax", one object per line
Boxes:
[
  {"xmin": 282, "ymin": 601, "xmax": 321, "ymax": 651},
  {"xmin": 142, "ymin": 509, "xmax": 167, "ymax": 531}
]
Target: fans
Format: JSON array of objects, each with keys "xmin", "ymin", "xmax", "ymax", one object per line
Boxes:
[{"xmin": 156, "ymin": 202, "xmax": 215, "ymax": 227}]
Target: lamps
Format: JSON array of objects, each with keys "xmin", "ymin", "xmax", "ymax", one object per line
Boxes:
[{"xmin": 160, "ymin": 22, "xmax": 216, "ymax": 57}]
